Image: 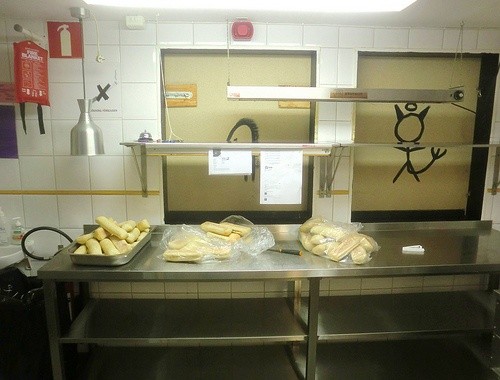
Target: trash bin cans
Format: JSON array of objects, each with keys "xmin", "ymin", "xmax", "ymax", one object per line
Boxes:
[{"xmin": 0, "ymin": 265, "xmax": 44, "ymax": 380}]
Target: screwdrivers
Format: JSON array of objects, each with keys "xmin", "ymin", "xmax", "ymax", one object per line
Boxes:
[{"xmin": 267, "ymin": 249, "xmax": 303, "ymax": 256}]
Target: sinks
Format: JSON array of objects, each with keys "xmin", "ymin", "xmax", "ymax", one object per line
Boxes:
[{"xmin": 0, "ymin": 244, "xmax": 34, "ymax": 270}]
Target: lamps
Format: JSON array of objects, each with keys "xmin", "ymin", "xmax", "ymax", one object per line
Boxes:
[{"xmin": 68, "ymin": 8, "xmax": 104, "ymax": 157}]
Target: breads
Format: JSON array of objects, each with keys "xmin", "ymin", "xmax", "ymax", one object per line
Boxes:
[{"xmin": 73, "ymin": 216, "xmax": 379, "ymax": 265}]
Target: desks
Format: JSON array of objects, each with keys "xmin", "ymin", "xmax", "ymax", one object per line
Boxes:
[{"xmin": 38, "ymin": 221, "xmax": 500, "ymax": 380}]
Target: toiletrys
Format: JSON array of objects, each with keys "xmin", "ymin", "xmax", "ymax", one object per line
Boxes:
[{"xmin": 8, "ymin": 217, "xmax": 27, "ymax": 245}]
